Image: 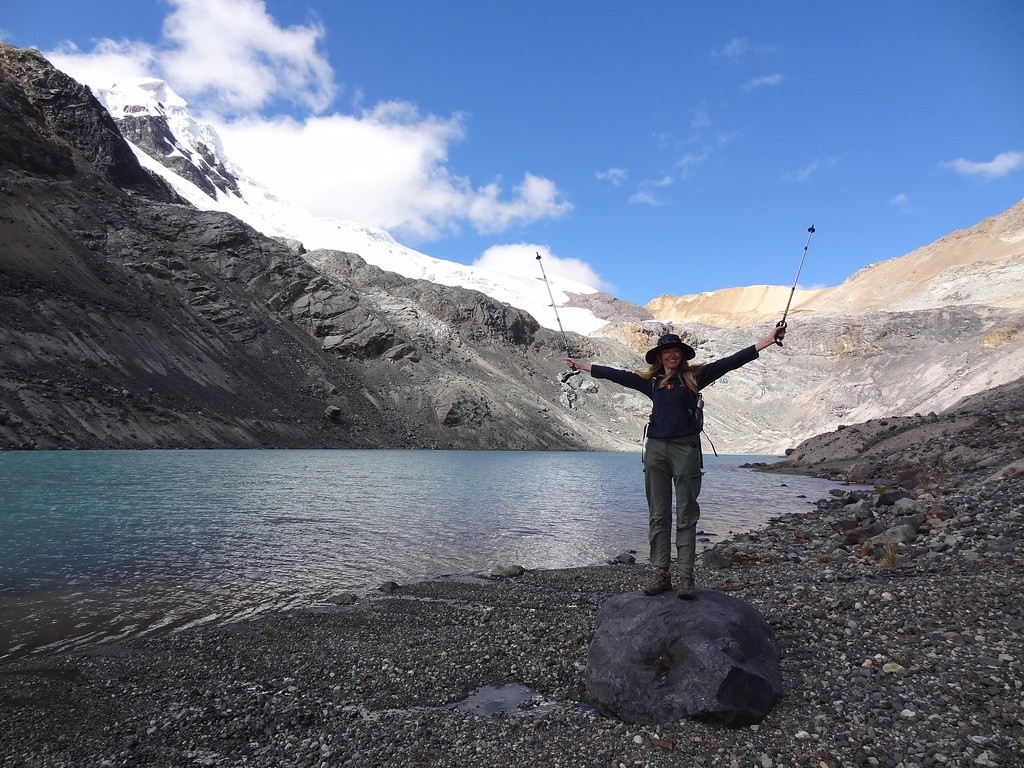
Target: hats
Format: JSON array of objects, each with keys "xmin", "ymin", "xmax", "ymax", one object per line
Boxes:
[{"xmin": 646, "ymin": 334, "xmax": 695, "ymax": 365}]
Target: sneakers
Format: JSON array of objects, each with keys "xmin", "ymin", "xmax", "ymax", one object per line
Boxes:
[
  {"xmin": 644, "ymin": 572, "xmax": 672, "ymax": 594},
  {"xmin": 677, "ymin": 570, "xmax": 695, "ymax": 598}
]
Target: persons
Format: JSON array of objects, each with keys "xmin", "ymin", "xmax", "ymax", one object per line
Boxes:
[{"xmin": 563, "ymin": 325, "xmax": 787, "ymax": 598}]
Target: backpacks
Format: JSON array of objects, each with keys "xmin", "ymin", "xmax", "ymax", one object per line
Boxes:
[{"xmin": 650, "ymin": 373, "xmax": 704, "ymax": 434}]
